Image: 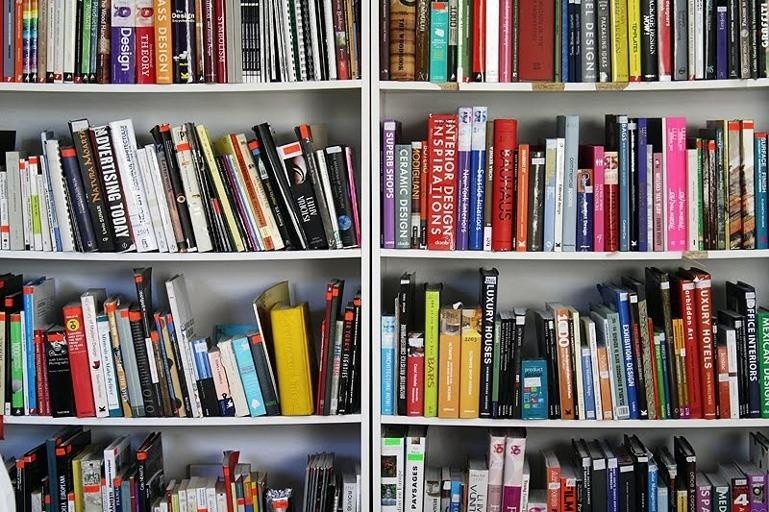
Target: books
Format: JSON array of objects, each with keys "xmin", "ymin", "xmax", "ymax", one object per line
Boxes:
[
  {"xmin": 381, "ymin": 428, "xmax": 769, "ymax": 512},
  {"xmin": 381, "ymin": 268, "xmax": 769, "ymax": 420},
  {"xmin": 0, "ymin": 268, "xmax": 361, "ymax": 418},
  {"xmin": 380, "ymin": 105, "xmax": 769, "ymax": 252},
  {"xmin": 1, "ymin": 2, "xmax": 364, "ymax": 82},
  {"xmin": 380, "ymin": 2, "xmax": 768, "ymax": 83},
  {"xmin": 1, "ymin": 426, "xmax": 361, "ymax": 512},
  {"xmin": 2, "ymin": 118, "xmax": 366, "ymax": 253}
]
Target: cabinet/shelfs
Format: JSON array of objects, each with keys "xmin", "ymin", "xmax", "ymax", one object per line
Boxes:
[
  {"xmin": 369, "ymin": 0, "xmax": 769, "ymax": 512},
  {"xmin": 0, "ymin": 0, "xmax": 371, "ymax": 512}
]
